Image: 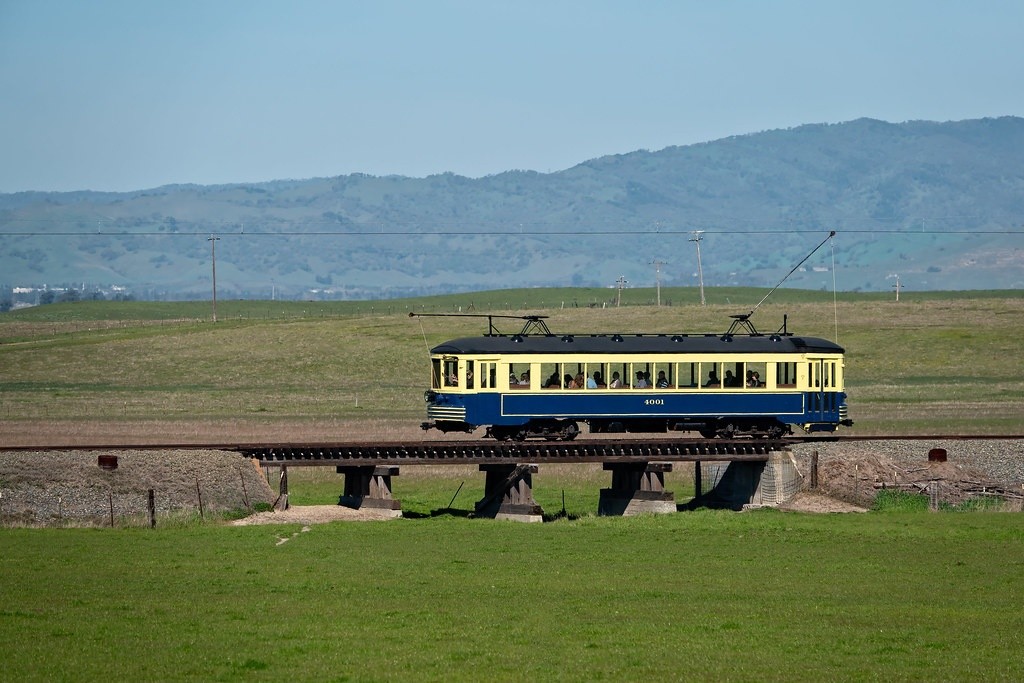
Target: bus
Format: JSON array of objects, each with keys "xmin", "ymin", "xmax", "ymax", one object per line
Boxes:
[{"xmin": 420, "ymin": 332, "xmax": 853, "ymax": 442}]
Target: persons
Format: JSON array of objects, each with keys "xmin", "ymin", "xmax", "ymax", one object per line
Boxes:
[
  {"xmin": 483, "ymin": 369, "xmax": 496, "ymax": 387},
  {"xmin": 610, "ymin": 372, "xmax": 622, "ymax": 388},
  {"xmin": 707, "ymin": 371, "xmax": 718, "ymax": 387},
  {"xmin": 545, "ymin": 371, "xmax": 607, "ymax": 388},
  {"xmin": 657, "ymin": 371, "xmax": 669, "ymax": 387},
  {"xmin": 724, "ymin": 370, "xmax": 740, "ymax": 387},
  {"xmin": 451, "ymin": 370, "xmax": 473, "ymax": 389},
  {"xmin": 746, "ymin": 371, "xmax": 761, "ymax": 387},
  {"xmin": 635, "ymin": 371, "xmax": 651, "ymax": 389},
  {"xmin": 509, "ymin": 370, "xmax": 530, "ymax": 384}
]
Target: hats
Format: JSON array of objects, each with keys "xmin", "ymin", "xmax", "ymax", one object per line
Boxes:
[
  {"xmin": 658, "ymin": 371, "xmax": 665, "ymax": 376},
  {"xmin": 635, "ymin": 370, "xmax": 644, "ymax": 376}
]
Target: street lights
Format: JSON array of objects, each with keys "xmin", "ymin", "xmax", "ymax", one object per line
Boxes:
[
  {"xmin": 208, "ymin": 237, "xmax": 221, "ymax": 324},
  {"xmin": 689, "ymin": 237, "xmax": 704, "ymax": 305}
]
